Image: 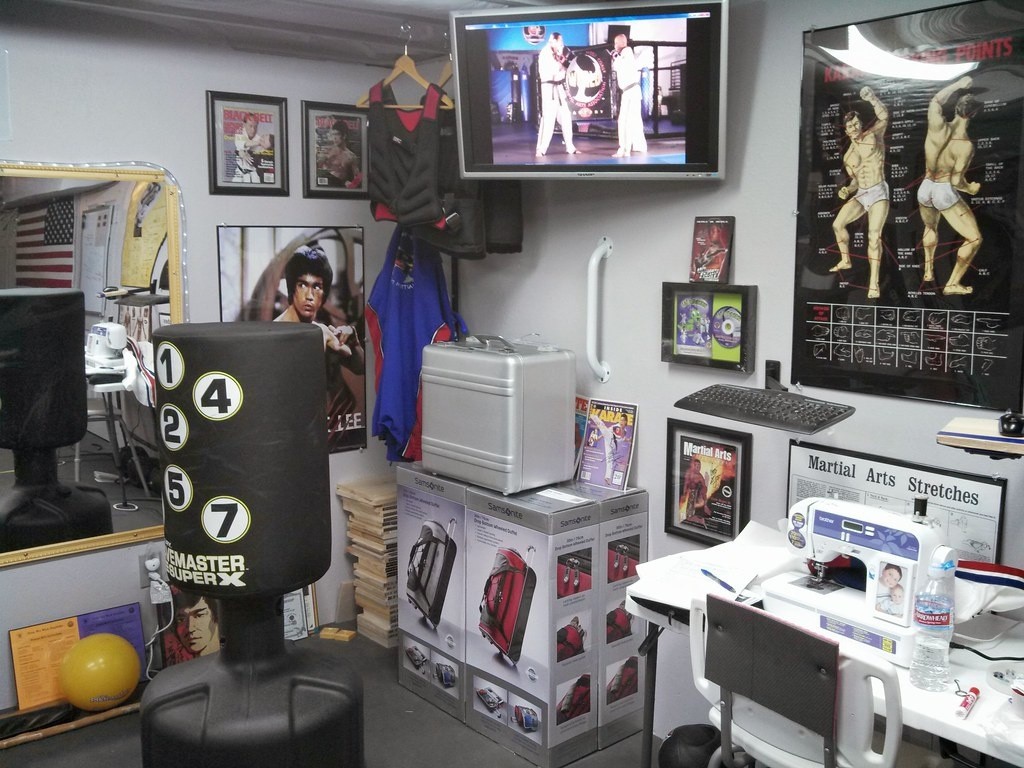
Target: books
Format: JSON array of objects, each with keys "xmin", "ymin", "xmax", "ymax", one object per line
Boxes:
[{"xmin": 336, "ymin": 477, "xmax": 398, "ymax": 649}]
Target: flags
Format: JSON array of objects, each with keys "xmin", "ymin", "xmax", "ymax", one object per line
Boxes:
[{"xmin": 15, "ymin": 197, "xmax": 74, "ymax": 289}]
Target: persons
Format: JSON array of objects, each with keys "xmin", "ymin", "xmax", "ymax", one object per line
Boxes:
[
  {"xmin": 324, "ymin": 122, "xmax": 360, "ymax": 188},
  {"xmin": 611, "ymin": 33, "xmax": 647, "ymax": 158},
  {"xmin": 233, "ymin": 113, "xmax": 273, "ymax": 183},
  {"xmin": 267, "ymin": 246, "xmax": 365, "ymax": 412},
  {"xmin": 591, "ymin": 413, "xmax": 632, "ymax": 485},
  {"xmin": 536, "ymin": 33, "xmax": 582, "ymax": 156},
  {"xmin": 877, "ymin": 564, "xmax": 904, "ymax": 618}
]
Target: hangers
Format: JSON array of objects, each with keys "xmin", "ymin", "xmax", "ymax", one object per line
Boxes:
[
  {"xmin": 436, "ymin": 32, "xmax": 454, "ymax": 89},
  {"xmin": 355, "ymin": 28, "xmax": 454, "ymax": 111}
]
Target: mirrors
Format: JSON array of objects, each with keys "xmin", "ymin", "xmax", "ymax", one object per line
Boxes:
[{"xmin": 0, "ymin": 163, "xmax": 184, "ymax": 569}]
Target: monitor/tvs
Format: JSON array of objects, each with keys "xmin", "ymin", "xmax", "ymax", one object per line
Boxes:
[{"xmin": 450, "ymin": 0, "xmax": 729, "ymax": 182}]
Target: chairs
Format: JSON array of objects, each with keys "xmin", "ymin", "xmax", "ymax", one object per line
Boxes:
[
  {"xmin": 71, "ymin": 397, "xmax": 151, "ymax": 499},
  {"xmin": 689, "ymin": 592, "xmax": 903, "ymax": 768}
]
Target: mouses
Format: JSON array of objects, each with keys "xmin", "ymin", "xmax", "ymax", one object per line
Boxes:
[
  {"xmin": 999, "ymin": 414, "xmax": 1023, "ymax": 436},
  {"xmin": 103, "ymin": 286, "xmax": 118, "ymax": 292}
]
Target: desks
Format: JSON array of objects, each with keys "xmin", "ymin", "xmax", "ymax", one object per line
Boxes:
[{"xmin": 624, "ymin": 521, "xmax": 1024, "ymax": 768}]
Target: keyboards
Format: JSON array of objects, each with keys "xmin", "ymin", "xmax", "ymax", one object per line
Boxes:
[{"xmin": 674, "ymin": 382, "xmax": 856, "ymax": 436}]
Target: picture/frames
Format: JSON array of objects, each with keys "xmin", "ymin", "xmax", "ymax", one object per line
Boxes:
[
  {"xmin": 303, "ymin": 583, "xmax": 320, "ymax": 636},
  {"xmin": 301, "ymin": 99, "xmax": 370, "ymax": 201},
  {"xmin": 665, "ymin": 417, "xmax": 753, "ymax": 545},
  {"xmin": 205, "ymin": 90, "xmax": 289, "ymax": 197}
]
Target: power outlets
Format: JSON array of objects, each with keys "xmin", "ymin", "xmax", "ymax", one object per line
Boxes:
[{"xmin": 138, "ymin": 552, "xmax": 162, "ymax": 589}]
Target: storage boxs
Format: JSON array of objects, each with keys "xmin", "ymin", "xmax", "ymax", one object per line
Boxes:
[{"xmin": 398, "ymin": 462, "xmax": 648, "ymax": 768}]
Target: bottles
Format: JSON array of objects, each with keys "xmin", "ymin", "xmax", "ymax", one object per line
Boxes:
[{"xmin": 910, "ymin": 564, "xmax": 954, "ymax": 693}]
